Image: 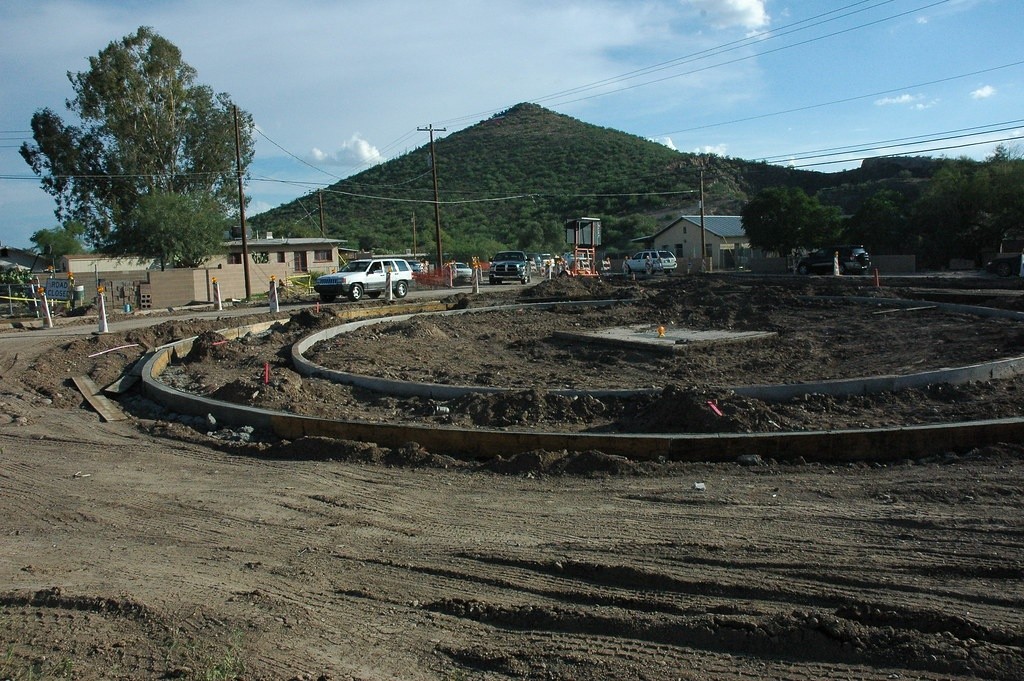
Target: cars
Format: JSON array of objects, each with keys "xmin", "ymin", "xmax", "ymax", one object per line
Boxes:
[
  {"xmin": 408, "ymin": 260, "xmax": 422, "ymax": 272},
  {"xmin": 448, "ymin": 262, "xmax": 472, "ymax": 278},
  {"xmin": 984, "ymin": 255, "xmax": 1023, "ymax": 277}
]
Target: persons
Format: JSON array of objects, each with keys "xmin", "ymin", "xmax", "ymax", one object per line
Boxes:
[
  {"xmin": 624, "ymin": 255, "xmax": 630, "ymax": 274},
  {"xmin": 556, "ymin": 256, "xmax": 566, "ymax": 274},
  {"xmin": 545, "ymin": 256, "xmax": 552, "ymax": 272},
  {"xmin": 645, "ymin": 256, "xmax": 652, "ymax": 274},
  {"xmin": 606, "ymin": 258, "xmax": 611, "ymax": 272}
]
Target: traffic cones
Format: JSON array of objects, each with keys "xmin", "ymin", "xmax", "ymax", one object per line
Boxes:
[
  {"xmin": 91, "ymin": 293, "xmax": 114, "ymax": 335},
  {"xmin": 36, "ymin": 295, "xmax": 58, "ymax": 332}
]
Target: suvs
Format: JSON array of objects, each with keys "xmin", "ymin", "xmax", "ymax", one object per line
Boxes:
[
  {"xmin": 622, "ymin": 250, "xmax": 677, "ymax": 274},
  {"xmin": 788, "ymin": 243, "xmax": 871, "ymax": 274},
  {"xmin": 314, "ymin": 258, "xmax": 414, "ymax": 302},
  {"xmin": 487, "ymin": 251, "xmax": 531, "ymax": 284},
  {"xmin": 526, "ymin": 253, "xmax": 584, "ymax": 270}
]
